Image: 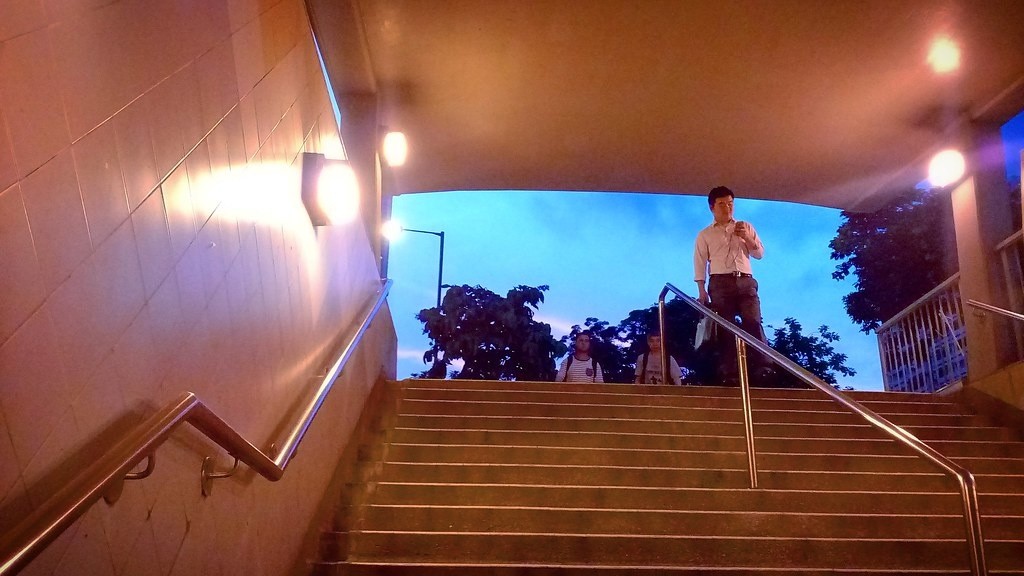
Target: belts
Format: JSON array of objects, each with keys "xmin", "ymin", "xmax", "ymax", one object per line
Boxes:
[{"xmin": 711, "ymin": 271, "xmax": 752, "ymax": 277}]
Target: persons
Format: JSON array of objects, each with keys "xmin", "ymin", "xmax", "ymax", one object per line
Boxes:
[
  {"xmin": 634, "ymin": 332, "xmax": 683, "ymax": 385},
  {"xmin": 555, "ymin": 333, "xmax": 603, "ymax": 383},
  {"xmin": 693, "ymin": 186, "xmax": 777, "ymax": 388}
]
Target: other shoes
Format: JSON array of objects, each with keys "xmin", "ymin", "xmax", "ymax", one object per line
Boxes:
[
  {"xmin": 722, "ymin": 377, "xmax": 739, "ymax": 386},
  {"xmin": 755, "ymin": 369, "xmax": 774, "ymax": 387}
]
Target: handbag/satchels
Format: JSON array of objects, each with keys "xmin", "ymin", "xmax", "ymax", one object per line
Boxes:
[{"xmin": 694, "ymin": 301, "xmax": 718, "ymax": 350}]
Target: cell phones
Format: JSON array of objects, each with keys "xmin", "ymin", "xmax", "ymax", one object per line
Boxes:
[{"xmin": 733, "ymin": 221, "xmax": 745, "ymax": 237}]
